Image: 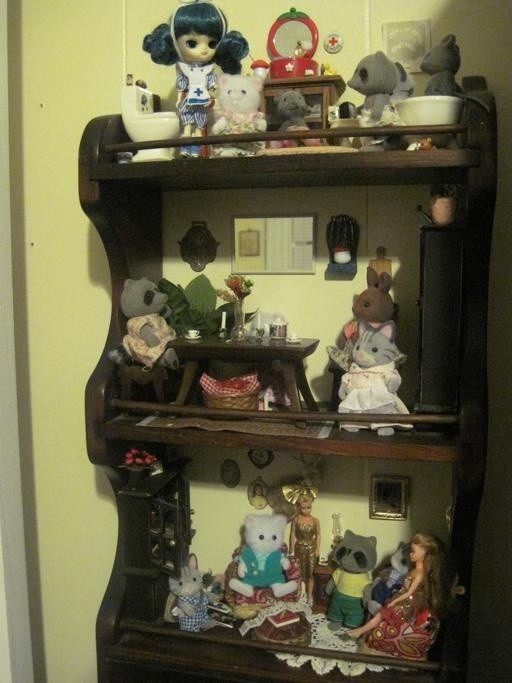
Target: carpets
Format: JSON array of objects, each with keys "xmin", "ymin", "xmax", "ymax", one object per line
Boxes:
[
  {"xmin": 134, "ymin": 399, "xmax": 339, "ymax": 440},
  {"xmin": 266, "ymin": 592, "xmax": 405, "ymax": 677}
]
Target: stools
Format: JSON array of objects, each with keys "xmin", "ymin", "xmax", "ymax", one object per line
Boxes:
[{"xmin": 117, "ymin": 360, "xmax": 171, "ymax": 416}]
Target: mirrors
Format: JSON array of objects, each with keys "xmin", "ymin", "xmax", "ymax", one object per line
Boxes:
[
  {"xmin": 229, "ymin": 210, "xmax": 321, "ymax": 278},
  {"xmin": 264, "ymin": 4, "xmax": 323, "ymax": 58}
]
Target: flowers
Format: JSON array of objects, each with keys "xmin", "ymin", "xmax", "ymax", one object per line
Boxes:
[
  {"xmin": 210, "ymin": 271, "xmax": 255, "ymax": 329},
  {"xmin": 117, "ymin": 444, "xmax": 158, "ymax": 472}
]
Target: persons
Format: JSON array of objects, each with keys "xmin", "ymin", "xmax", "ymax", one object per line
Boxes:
[
  {"xmin": 143, "ymin": 0, "xmax": 248, "ymax": 157},
  {"xmin": 252, "ymin": 482, "xmax": 268, "ymax": 509},
  {"xmin": 345, "ymin": 532, "xmax": 447, "ymax": 642},
  {"xmin": 288, "ymin": 474, "xmax": 320, "ymax": 607}
]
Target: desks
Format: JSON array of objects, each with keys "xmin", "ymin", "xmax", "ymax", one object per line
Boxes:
[
  {"xmin": 164, "ymin": 327, "xmax": 325, "ymax": 432},
  {"xmin": 257, "ymin": 73, "xmax": 349, "ymax": 146}
]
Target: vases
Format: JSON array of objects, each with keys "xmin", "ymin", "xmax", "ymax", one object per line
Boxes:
[
  {"xmin": 226, "ymin": 298, "xmax": 248, "ymax": 343},
  {"xmin": 119, "ymin": 463, "xmax": 146, "ymax": 483}
]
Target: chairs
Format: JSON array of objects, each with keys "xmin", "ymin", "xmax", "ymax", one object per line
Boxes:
[
  {"xmin": 320, "ymin": 302, "xmax": 403, "ymax": 410},
  {"xmin": 222, "ymin": 541, "xmax": 305, "ymax": 614},
  {"xmin": 360, "ymin": 570, "xmax": 461, "ymax": 663}
]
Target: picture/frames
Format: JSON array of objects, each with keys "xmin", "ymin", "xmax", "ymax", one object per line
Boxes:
[
  {"xmin": 368, "ymin": 473, "xmax": 411, "ymax": 524},
  {"xmin": 237, "ymin": 226, "xmax": 263, "ymax": 257},
  {"xmin": 218, "ymin": 446, "xmax": 275, "ymax": 510}
]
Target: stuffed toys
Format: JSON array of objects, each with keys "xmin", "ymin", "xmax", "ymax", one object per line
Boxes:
[
  {"xmin": 346, "ymin": 31, "xmax": 465, "ymax": 149},
  {"xmin": 325, "ymin": 528, "xmax": 378, "ymax": 628},
  {"xmin": 119, "ymin": 277, "xmax": 182, "ymax": 370},
  {"xmin": 228, "ymin": 510, "xmax": 299, "ymax": 600},
  {"xmin": 325, "ymin": 318, "xmax": 404, "ymax": 436},
  {"xmin": 334, "ymin": 264, "xmax": 396, "ymax": 351},
  {"xmin": 211, "ymin": 71, "xmax": 269, "ymax": 160},
  {"xmin": 271, "ymin": 87, "xmax": 320, "ymax": 148},
  {"xmin": 363, "ymin": 540, "xmax": 416, "ymax": 617},
  {"xmin": 166, "ymin": 553, "xmax": 224, "ymax": 632}
]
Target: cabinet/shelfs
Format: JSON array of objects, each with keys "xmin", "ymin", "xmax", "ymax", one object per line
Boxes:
[
  {"xmin": 71, "ymin": 70, "xmax": 499, "ymax": 682},
  {"xmin": 413, "ymin": 220, "xmax": 471, "ymax": 432},
  {"xmin": 116, "ymin": 454, "xmax": 192, "ymax": 623},
  {"xmin": 312, "ymin": 559, "xmax": 340, "ymax": 616}
]
